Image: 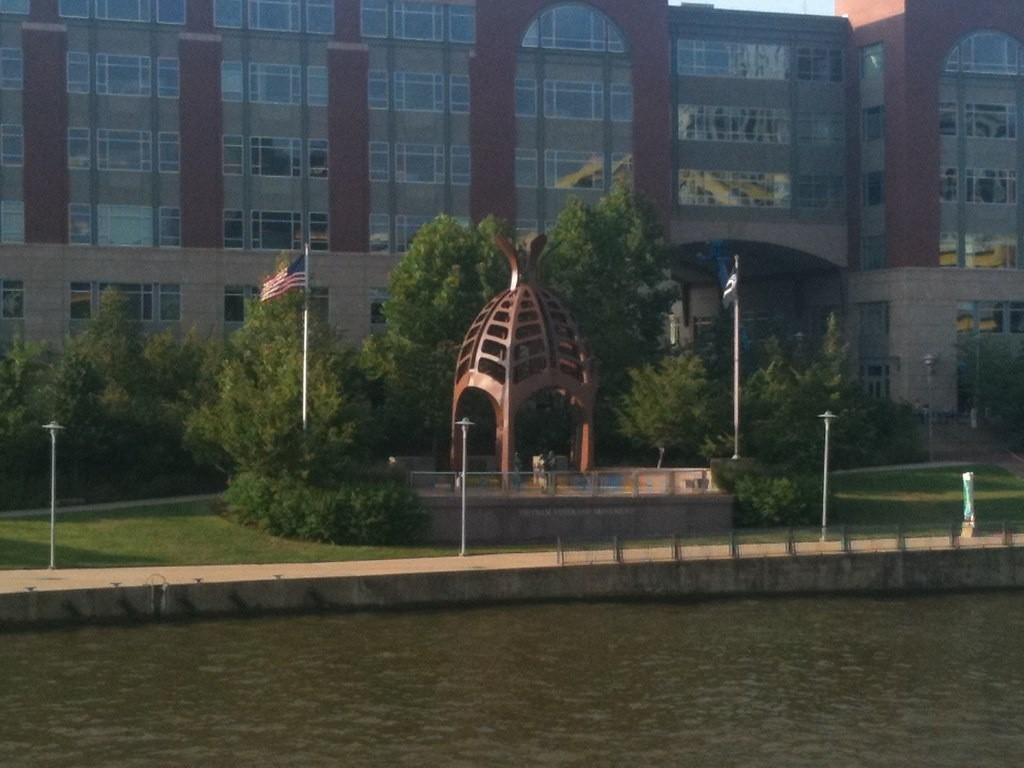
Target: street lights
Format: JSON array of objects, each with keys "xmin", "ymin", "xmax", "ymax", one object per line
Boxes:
[
  {"xmin": 923, "ymin": 353, "xmax": 937, "ymax": 463},
  {"xmin": 817, "ymin": 410, "xmax": 838, "ymax": 542},
  {"xmin": 42, "ymin": 419, "xmax": 68, "ymax": 571},
  {"xmin": 453, "ymin": 417, "xmax": 476, "ymax": 556}
]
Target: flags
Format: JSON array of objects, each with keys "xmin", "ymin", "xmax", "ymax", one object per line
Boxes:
[{"xmin": 259, "ymin": 252, "xmax": 307, "ymax": 302}]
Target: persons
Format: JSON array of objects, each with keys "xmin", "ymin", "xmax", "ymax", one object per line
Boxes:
[{"xmin": 511, "ymin": 450, "xmax": 557, "ymax": 490}]
